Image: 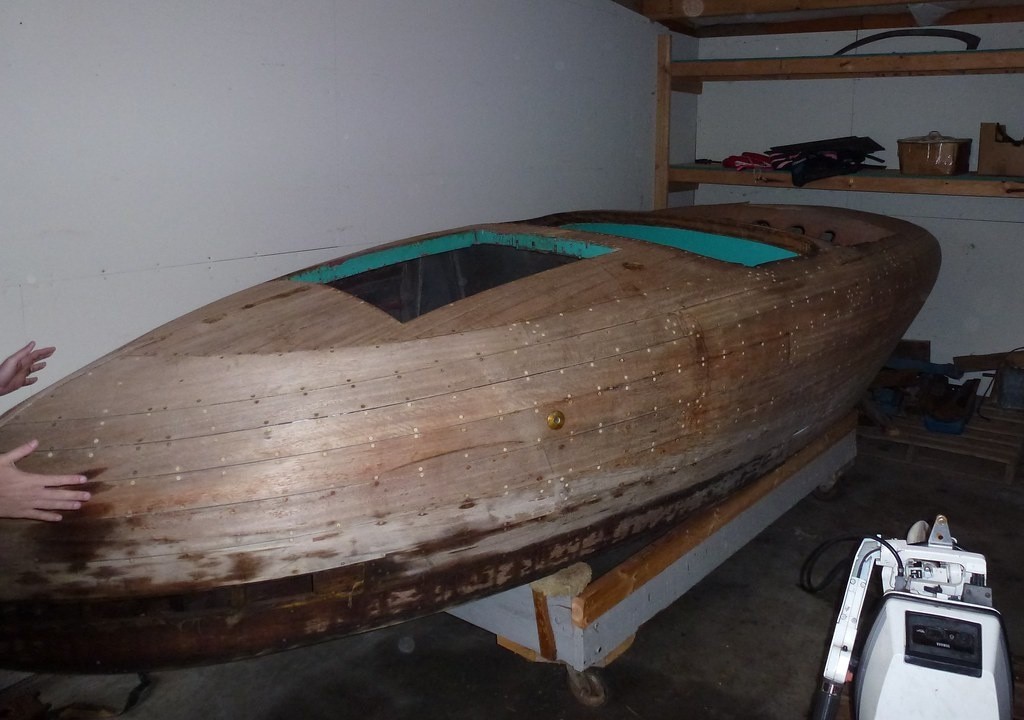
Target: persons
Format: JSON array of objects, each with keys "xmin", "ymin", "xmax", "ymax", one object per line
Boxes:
[{"xmin": 0, "ymin": 341, "xmax": 91, "ymax": 522}]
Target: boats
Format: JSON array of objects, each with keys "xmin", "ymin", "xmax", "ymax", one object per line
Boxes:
[{"xmin": 0, "ymin": 204, "xmax": 942, "ymax": 673}]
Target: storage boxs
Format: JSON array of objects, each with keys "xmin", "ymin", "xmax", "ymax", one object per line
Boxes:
[
  {"xmin": 977, "ymin": 122, "xmax": 1024, "ymax": 177},
  {"xmin": 896, "ymin": 130, "xmax": 973, "ymax": 176}
]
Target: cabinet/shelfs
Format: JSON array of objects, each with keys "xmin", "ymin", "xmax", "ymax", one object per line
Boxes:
[{"xmin": 656, "ymin": 34, "xmax": 1024, "ymax": 209}]
